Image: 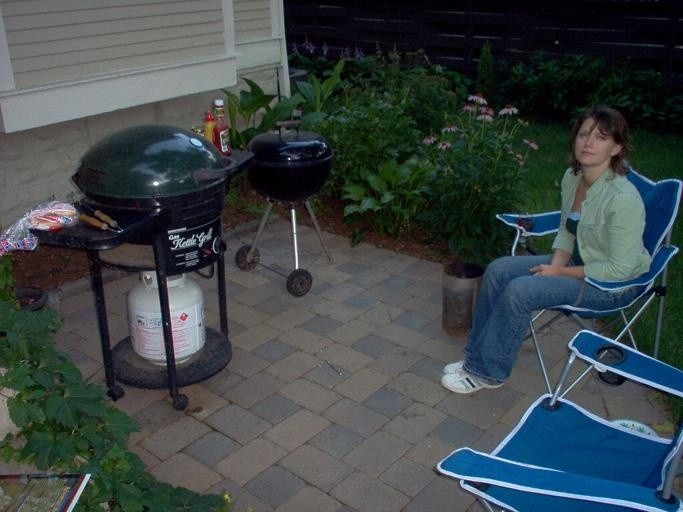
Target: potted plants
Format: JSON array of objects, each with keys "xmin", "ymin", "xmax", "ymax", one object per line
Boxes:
[{"xmin": 441, "ymin": 264, "xmax": 486, "ymax": 341}]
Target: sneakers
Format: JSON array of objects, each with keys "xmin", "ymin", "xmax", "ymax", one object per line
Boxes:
[{"xmin": 441, "ymin": 359, "xmax": 504, "ymax": 394}]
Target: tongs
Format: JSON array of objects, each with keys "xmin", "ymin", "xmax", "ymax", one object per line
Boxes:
[{"xmin": 52, "ymin": 191, "xmax": 124, "ymax": 235}]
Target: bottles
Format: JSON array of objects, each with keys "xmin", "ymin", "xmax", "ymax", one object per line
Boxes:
[
  {"xmin": 214, "ymin": 98, "xmax": 232, "ymax": 156},
  {"xmin": 205, "ymin": 111, "xmax": 216, "ymax": 145}
]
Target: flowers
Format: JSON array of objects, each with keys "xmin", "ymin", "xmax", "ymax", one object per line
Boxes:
[{"xmin": 423, "ymin": 94, "xmax": 539, "ymax": 278}]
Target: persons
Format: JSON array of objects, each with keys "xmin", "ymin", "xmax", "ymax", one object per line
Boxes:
[{"xmin": 440, "ymin": 106, "xmax": 654, "ymax": 397}]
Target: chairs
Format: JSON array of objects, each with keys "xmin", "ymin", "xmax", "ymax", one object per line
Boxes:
[
  {"xmin": 433, "ymin": 329, "xmax": 683, "ymax": 511},
  {"xmin": 494, "ymin": 166, "xmax": 683, "ymax": 398}
]
